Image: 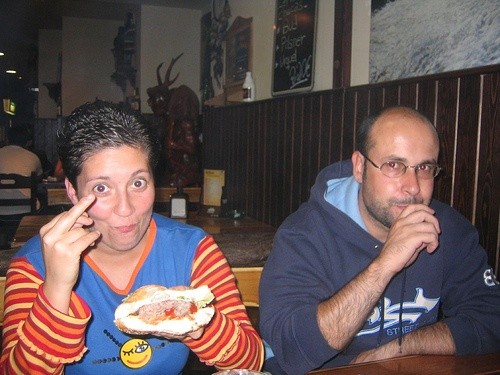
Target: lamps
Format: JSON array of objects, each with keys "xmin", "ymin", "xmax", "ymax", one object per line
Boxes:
[{"xmin": 3, "ymin": 99, "xmax": 16, "ymax": 116}]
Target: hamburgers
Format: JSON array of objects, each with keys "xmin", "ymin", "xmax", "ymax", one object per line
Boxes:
[{"xmin": 113, "ymin": 284, "xmax": 216, "ymax": 335}]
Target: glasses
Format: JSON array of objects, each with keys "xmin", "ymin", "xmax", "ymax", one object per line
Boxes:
[{"xmin": 364, "ymin": 155, "xmax": 443, "ymax": 178}]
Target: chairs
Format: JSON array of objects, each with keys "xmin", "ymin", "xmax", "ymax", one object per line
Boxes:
[
  {"xmin": 0, "ymin": 172, "xmax": 49, "ymax": 248},
  {"xmin": 178, "ymin": 306, "xmax": 268, "ymax": 374}
]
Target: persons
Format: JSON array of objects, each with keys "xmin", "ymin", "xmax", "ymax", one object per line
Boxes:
[
  {"xmin": 259, "ymin": 103, "xmax": 500, "ymax": 375},
  {"xmin": 0, "ymin": 100, "xmax": 265, "ymax": 375}
]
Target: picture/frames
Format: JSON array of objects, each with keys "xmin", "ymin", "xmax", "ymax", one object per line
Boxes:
[
  {"xmin": 224, "ymin": 18, "xmax": 254, "ymax": 90},
  {"xmin": 271, "ymin": 0, "xmax": 319, "ymax": 97}
]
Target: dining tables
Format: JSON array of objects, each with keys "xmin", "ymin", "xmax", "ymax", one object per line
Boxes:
[
  {"xmin": 288, "ymin": 354, "xmax": 500, "ymax": 375},
  {"xmin": 13, "ymin": 214, "xmax": 285, "ymax": 335},
  {"xmin": 41, "ymin": 177, "xmax": 203, "ymax": 220}
]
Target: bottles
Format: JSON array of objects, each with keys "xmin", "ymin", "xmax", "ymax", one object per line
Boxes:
[
  {"xmin": 242, "ymin": 72, "xmax": 255, "ymax": 101},
  {"xmin": 170, "ymin": 185, "xmax": 189, "ymax": 219}
]
